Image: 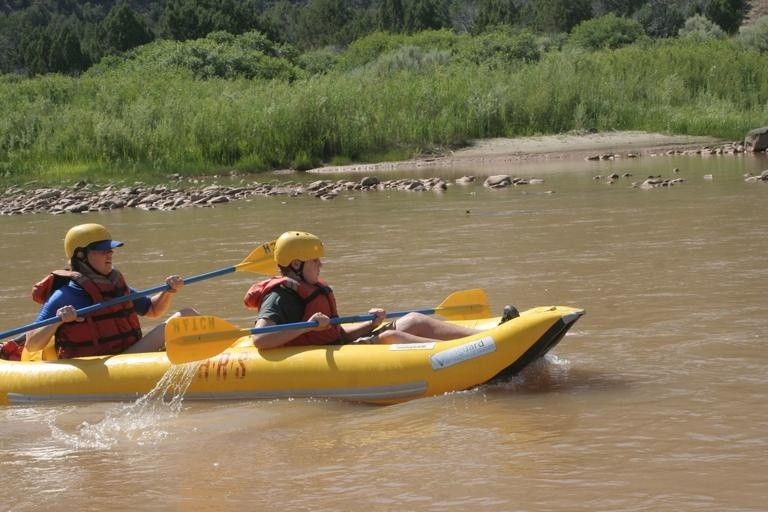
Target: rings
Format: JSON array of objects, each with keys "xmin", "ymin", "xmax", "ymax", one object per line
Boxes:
[{"xmin": 170, "ymin": 279, "xmax": 174, "ymax": 284}]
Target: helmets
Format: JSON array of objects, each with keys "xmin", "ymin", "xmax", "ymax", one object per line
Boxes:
[
  {"xmin": 63, "ymin": 224, "xmax": 123, "ymax": 258},
  {"xmin": 274, "ymin": 231, "xmax": 327, "ymax": 268}
]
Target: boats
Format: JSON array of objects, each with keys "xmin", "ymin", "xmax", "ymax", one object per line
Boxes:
[{"xmin": 1, "ymin": 303, "xmax": 586, "ymax": 409}]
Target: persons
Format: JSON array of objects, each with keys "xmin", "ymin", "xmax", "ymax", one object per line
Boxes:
[
  {"xmin": 250, "ymin": 229, "xmax": 520, "ymax": 350},
  {"xmin": 25, "ymin": 221, "xmax": 203, "ymax": 355}
]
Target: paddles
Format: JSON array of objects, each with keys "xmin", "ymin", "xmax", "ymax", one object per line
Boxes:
[
  {"xmin": 164, "ymin": 287, "xmax": 494, "ymax": 364},
  {"xmin": 0, "ymin": 240, "xmax": 275, "ymax": 341}
]
